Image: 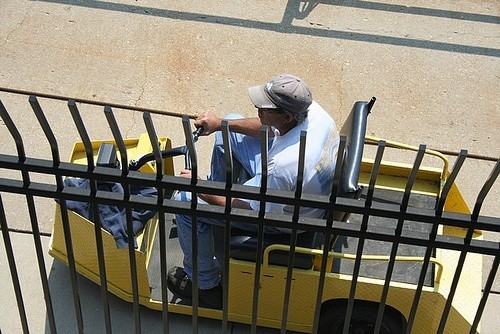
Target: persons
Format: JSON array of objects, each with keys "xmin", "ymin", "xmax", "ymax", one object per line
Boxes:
[{"xmin": 166, "ymin": 75, "xmax": 342, "ymax": 310}]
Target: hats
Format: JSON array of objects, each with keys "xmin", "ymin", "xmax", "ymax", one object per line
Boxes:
[{"xmin": 247, "ymin": 73, "xmax": 313, "ymax": 117}]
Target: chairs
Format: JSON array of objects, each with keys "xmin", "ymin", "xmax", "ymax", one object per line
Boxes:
[{"xmin": 228, "ymin": 95, "xmax": 376, "ymax": 270}]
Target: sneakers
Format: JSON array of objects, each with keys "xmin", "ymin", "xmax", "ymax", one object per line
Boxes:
[{"xmin": 167, "ymin": 264, "xmax": 224, "ymax": 309}]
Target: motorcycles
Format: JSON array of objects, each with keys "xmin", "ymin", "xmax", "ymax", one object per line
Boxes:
[{"xmin": 48, "ymin": 96, "xmax": 483, "ymax": 334}]
